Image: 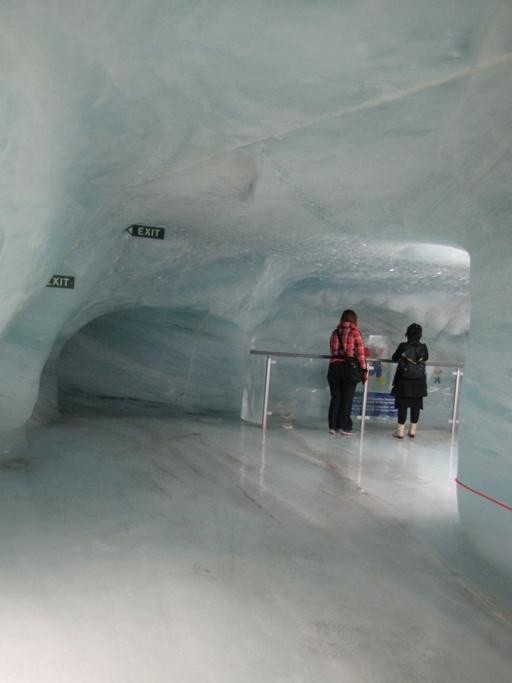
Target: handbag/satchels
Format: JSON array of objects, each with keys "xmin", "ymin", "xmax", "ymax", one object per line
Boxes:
[{"xmin": 345, "ymin": 358, "xmax": 363, "ymax": 381}]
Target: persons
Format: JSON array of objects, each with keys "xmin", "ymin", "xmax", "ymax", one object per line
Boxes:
[
  {"xmin": 392, "ymin": 322, "xmax": 429, "ymax": 439},
  {"xmin": 328, "ymin": 309, "xmax": 367, "ymax": 435}
]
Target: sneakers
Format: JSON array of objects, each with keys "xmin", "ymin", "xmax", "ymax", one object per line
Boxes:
[{"xmin": 329, "ymin": 429, "xmax": 356, "ymax": 436}]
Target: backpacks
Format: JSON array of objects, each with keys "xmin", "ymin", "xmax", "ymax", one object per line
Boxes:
[{"xmin": 398, "ymin": 348, "xmax": 426, "ymax": 379}]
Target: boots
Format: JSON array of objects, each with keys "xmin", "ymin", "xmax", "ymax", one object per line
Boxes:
[{"xmin": 392, "ymin": 424, "xmax": 417, "ymax": 439}]
